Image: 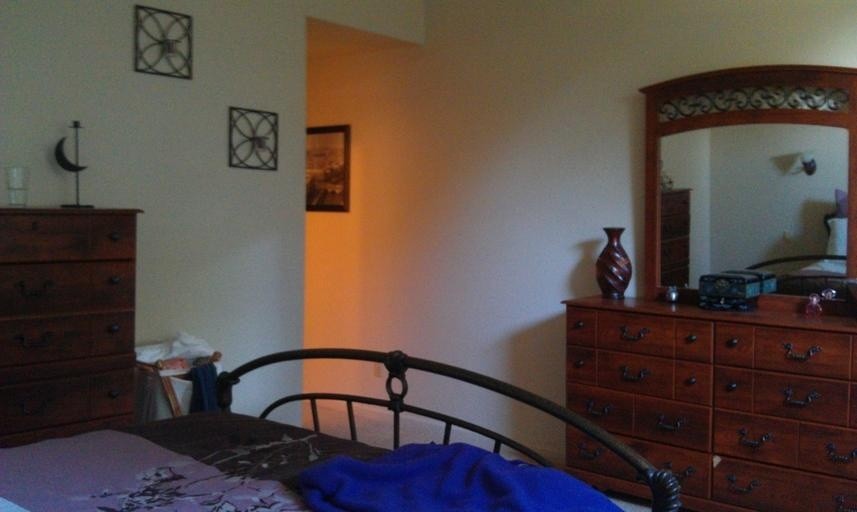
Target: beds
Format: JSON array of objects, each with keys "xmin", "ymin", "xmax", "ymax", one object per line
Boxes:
[
  {"xmin": 1, "ymin": 347, "xmax": 679, "ymax": 512},
  {"xmin": 744, "ymin": 189, "xmax": 847, "ymax": 297}
]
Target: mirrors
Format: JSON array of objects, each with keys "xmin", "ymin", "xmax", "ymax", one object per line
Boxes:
[{"xmin": 637, "ymin": 65, "xmax": 857, "ymax": 306}]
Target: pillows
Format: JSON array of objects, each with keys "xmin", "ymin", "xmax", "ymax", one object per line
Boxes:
[{"xmin": 824, "ymin": 190, "xmax": 850, "ymax": 257}]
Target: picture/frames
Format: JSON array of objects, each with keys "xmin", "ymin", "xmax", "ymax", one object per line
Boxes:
[{"xmin": 305, "ymin": 122, "xmax": 353, "ymax": 213}]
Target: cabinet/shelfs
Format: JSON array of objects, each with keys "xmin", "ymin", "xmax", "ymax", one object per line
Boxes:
[
  {"xmin": 659, "ymin": 187, "xmax": 693, "ymax": 285},
  {"xmin": 1, "ymin": 205, "xmax": 148, "ymax": 451},
  {"xmin": 557, "ymin": 291, "xmax": 857, "ymax": 512}
]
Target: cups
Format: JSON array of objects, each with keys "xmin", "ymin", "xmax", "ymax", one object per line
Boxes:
[{"xmin": 5, "ymin": 167, "xmax": 29, "ymax": 209}]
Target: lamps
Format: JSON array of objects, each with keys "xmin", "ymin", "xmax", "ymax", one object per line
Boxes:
[{"xmin": 798, "ymin": 152, "xmax": 818, "ymax": 175}]
